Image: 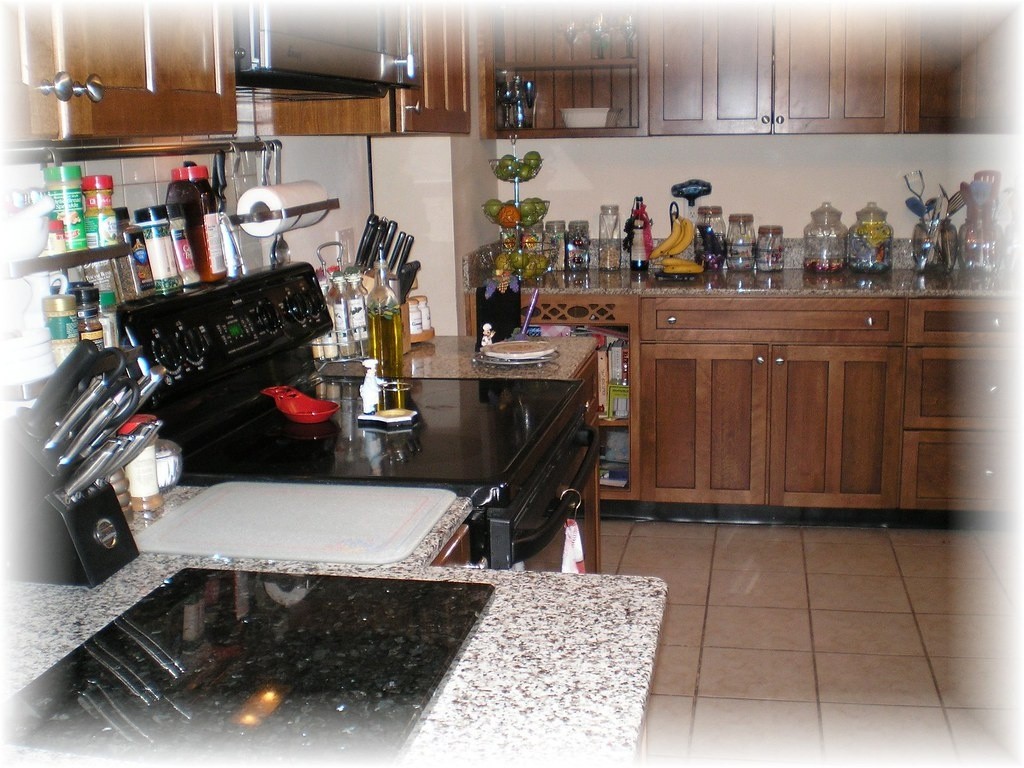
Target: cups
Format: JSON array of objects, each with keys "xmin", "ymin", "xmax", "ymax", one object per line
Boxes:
[{"xmin": 514, "ymin": 81, "xmax": 538, "ymax": 128}]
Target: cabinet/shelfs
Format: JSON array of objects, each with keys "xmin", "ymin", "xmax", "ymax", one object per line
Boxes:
[
  {"xmin": 8, "ymin": 3, "xmax": 242, "ymax": 165},
  {"xmin": 269, "ymin": 2, "xmax": 471, "ymax": 137},
  {"xmin": 643, "ymin": 2, "xmax": 914, "ymax": 134},
  {"xmin": 492, "ymin": 2, "xmax": 645, "ymax": 137},
  {"xmin": 464, "ymin": 288, "xmax": 640, "ymax": 505},
  {"xmin": 903, "ymin": 4, "xmax": 1020, "ymax": 133},
  {"xmin": 626, "ymin": 299, "xmax": 903, "ymax": 505},
  {"xmin": 572, "ymin": 350, "xmax": 600, "ymax": 576},
  {"xmin": 899, "ymin": 299, "xmax": 1017, "ymax": 509}
]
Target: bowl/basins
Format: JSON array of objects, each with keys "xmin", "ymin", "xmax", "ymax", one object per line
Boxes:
[{"xmin": 563, "ymin": 108, "xmax": 610, "ymax": 128}]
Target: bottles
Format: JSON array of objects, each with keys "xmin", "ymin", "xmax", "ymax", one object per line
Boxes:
[
  {"xmin": 36, "ymin": 163, "xmax": 225, "ymax": 370},
  {"xmin": 628, "ymin": 196, "xmax": 650, "ymax": 271},
  {"xmin": 846, "ymin": 202, "xmax": 894, "ymax": 272},
  {"xmin": 599, "ymin": 205, "xmax": 621, "ymax": 269},
  {"xmin": 803, "ymin": 202, "xmax": 848, "ymax": 272},
  {"xmin": 913, "ymin": 218, "xmax": 957, "ymax": 271},
  {"xmin": 756, "ymin": 225, "xmax": 784, "ymax": 272},
  {"xmin": 957, "ymin": 220, "xmax": 1004, "ymax": 271},
  {"xmin": 500, "ymin": 220, "xmax": 589, "ymax": 269},
  {"xmin": 694, "ymin": 205, "xmax": 726, "ymax": 269},
  {"xmin": 307, "ymin": 262, "xmax": 430, "ymax": 367},
  {"xmin": 727, "ymin": 214, "xmax": 756, "ymax": 271}
]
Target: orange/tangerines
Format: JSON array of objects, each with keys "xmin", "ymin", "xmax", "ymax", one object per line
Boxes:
[{"xmin": 484, "ymin": 152, "xmax": 551, "ymax": 278}]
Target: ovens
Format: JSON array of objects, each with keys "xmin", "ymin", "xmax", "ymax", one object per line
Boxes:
[{"xmin": 472, "ymin": 394, "xmax": 601, "ymax": 578}]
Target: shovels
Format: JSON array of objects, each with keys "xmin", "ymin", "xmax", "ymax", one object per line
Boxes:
[{"xmin": 904, "ymin": 170, "xmax": 1017, "ymax": 275}]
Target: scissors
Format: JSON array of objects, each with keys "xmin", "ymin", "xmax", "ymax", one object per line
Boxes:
[{"xmin": 60, "ymin": 347, "xmax": 141, "ymax": 433}]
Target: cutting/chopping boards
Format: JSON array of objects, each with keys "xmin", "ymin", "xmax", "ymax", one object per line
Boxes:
[{"xmin": 0, "ymin": 559, "xmax": 494, "ymax": 765}]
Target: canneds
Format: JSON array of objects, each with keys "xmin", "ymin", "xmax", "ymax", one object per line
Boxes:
[{"xmin": 42, "ymin": 166, "xmax": 196, "ymax": 371}]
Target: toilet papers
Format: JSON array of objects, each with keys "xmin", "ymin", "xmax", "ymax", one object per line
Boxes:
[{"xmin": 236, "ymin": 180, "xmax": 327, "ymax": 238}]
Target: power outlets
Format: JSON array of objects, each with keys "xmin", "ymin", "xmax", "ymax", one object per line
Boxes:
[{"xmin": 336, "ymin": 227, "xmax": 356, "ymax": 265}]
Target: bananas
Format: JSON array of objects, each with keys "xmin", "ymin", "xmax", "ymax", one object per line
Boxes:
[
  {"xmin": 653, "ymin": 258, "xmax": 705, "ymax": 273},
  {"xmin": 649, "ymin": 211, "xmax": 694, "ymax": 259}
]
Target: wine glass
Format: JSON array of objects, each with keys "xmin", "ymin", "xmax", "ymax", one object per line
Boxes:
[
  {"xmin": 562, "ymin": 11, "xmax": 636, "ymax": 61},
  {"xmin": 497, "ymin": 71, "xmax": 522, "ymax": 127}
]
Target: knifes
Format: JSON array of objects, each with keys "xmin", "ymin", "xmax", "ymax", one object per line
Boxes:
[
  {"xmin": 357, "ymin": 213, "xmax": 420, "ymax": 306},
  {"xmin": 21, "ymin": 342, "xmax": 167, "ymax": 496}
]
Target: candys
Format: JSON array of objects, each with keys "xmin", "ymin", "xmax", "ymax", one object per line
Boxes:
[
  {"xmin": 695, "ymin": 235, "xmax": 785, "ymax": 271},
  {"xmin": 806, "ymin": 223, "xmax": 893, "ymax": 273}
]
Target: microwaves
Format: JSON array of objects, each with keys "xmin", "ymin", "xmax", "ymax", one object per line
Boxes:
[{"xmin": 235, "ymin": 0, "xmax": 421, "ymax": 93}]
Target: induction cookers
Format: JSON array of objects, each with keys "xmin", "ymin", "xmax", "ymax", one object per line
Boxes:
[{"xmin": 117, "ymin": 264, "xmax": 584, "ymax": 503}]
